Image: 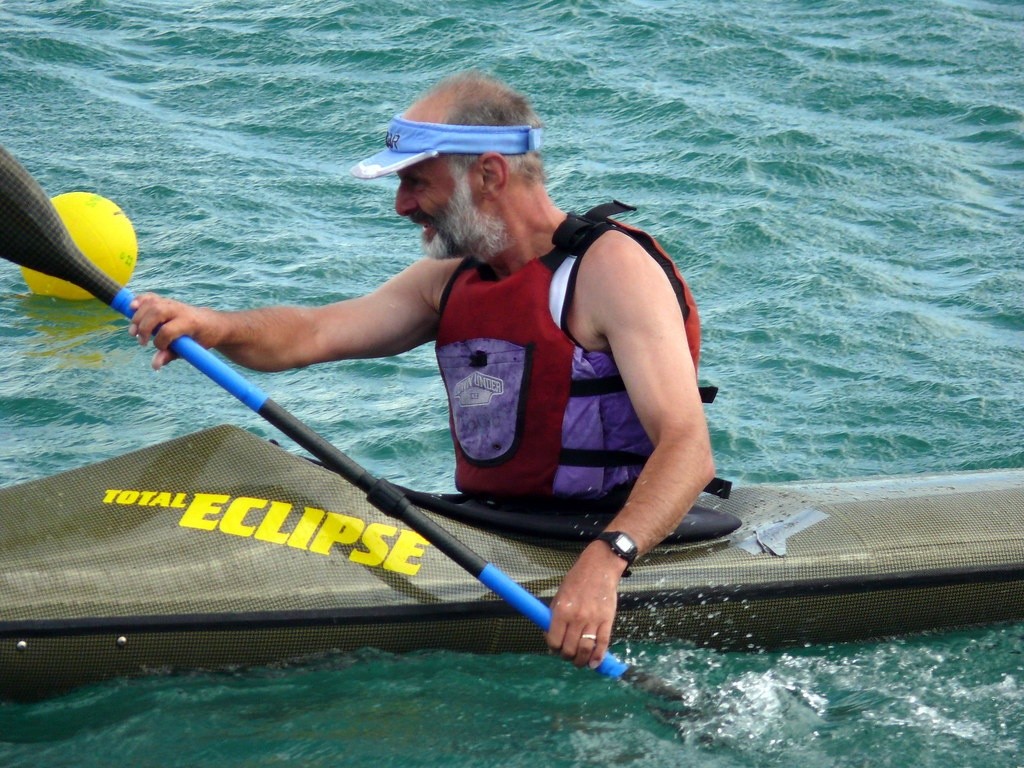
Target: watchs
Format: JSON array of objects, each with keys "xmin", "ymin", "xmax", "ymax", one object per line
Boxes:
[{"xmin": 598, "ymin": 531, "xmax": 637, "ymax": 578}]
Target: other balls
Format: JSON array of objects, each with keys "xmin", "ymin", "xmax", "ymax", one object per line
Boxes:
[{"xmin": 20, "ymin": 190, "xmax": 138, "ymax": 301}]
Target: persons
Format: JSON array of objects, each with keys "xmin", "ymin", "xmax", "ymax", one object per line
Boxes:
[{"xmin": 129, "ymin": 75, "xmax": 717, "ymax": 672}]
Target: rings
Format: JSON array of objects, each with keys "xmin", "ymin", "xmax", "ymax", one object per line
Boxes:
[{"xmin": 582, "ymin": 634, "xmax": 596, "ymax": 639}]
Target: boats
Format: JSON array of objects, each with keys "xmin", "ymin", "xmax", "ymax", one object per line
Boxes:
[{"xmin": 0, "ymin": 422, "xmax": 1024, "ymax": 697}]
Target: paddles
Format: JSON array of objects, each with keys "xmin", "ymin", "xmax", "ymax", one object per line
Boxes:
[{"xmin": 0, "ymin": 147, "xmax": 679, "ymax": 700}]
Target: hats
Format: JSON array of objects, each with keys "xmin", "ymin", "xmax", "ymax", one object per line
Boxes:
[{"xmin": 349, "ymin": 114, "xmax": 542, "ymax": 179}]
